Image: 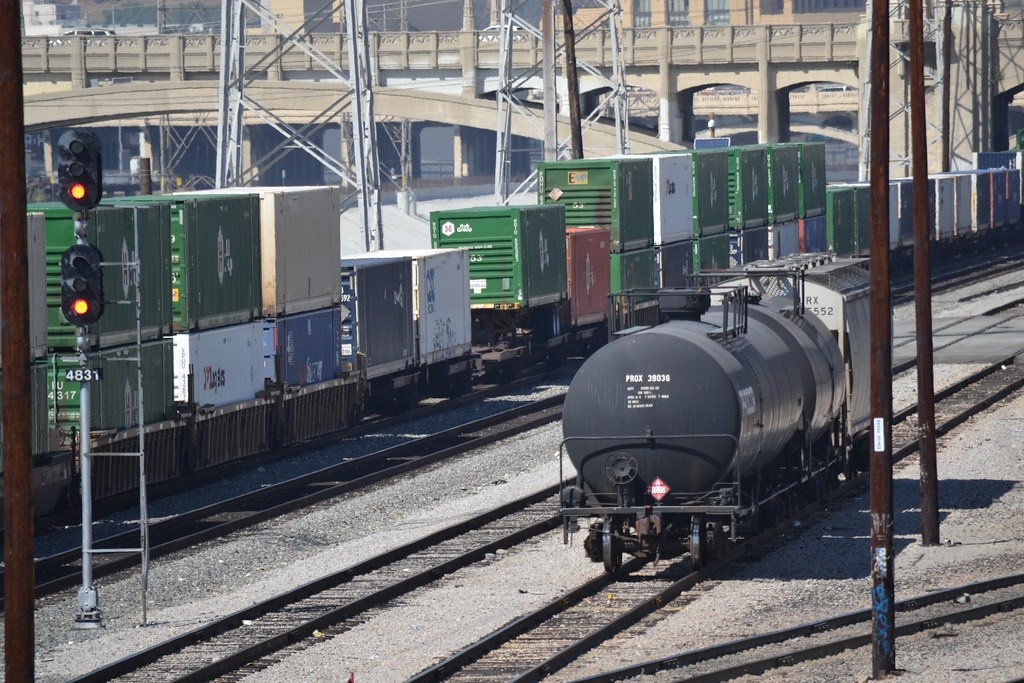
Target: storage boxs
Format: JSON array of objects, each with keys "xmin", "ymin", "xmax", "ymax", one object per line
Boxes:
[
  {"xmin": 799, "ymin": 142, "xmax": 827, "ymax": 217},
  {"xmin": 767, "ymin": 220, "xmax": 798, "ymax": 259},
  {"xmin": 539, "ymin": 158, "xmax": 653, "ymax": 251},
  {"xmin": 566, "ymin": 228, "xmax": 609, "ymax": 326},
  {"xmin": 430, "ymin": 205, "xmax": 567, "ymax": 309},
  {"xmin": 341, "ymin": 248, "xmax": 472, "ymax": 368},
  {"xmin": 653, "ymin": 152, "xmax": 693, "ymax": 246},
  {"xmin": 729, "ymin": 147, "xmax": 768, "ymax": 230},
  {"xmin": 765, "ymin": 145, "xmax": 799, "ymax": 224},
  {"xmin": 730, "ymin": 227, "xmax": 767, "ymax": 267},
  {"xmin": 693, "ymin": 149, "xmax": 728, "ymax": 235},
  {"xmin": 653, "ymin": 240, "xmax": 692, "ymax": 287},
  {"xmin": 799, "ymin": 215, "xmax": 828, "ymax": 255},
  {"xmin": 610, "ymin": 248, "xmax": 653, "ymax": 293},
  {"xmin": 826, "ymin": 170, "xmax": 1020, "ymax": 257},
  {"xmin": 28, "ymin": 186, "xmax": 340, "ymax": 454},
  {"xmin": 693, "ymin": 232, "xmax": 729, "ymax": 274}
]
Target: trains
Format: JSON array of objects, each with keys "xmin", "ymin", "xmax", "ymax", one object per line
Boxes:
[
  {"xmin": 558, "ymin": 251, "xmax": 878, "ymax": 576},
  {"xmin": 22, "ymin": 137, "xmax": 1024, "ymax": 538}
]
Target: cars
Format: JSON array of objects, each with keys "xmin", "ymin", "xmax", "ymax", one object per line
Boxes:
[{"xmin": 478, "ymin": 24, "xmax": 526, "ymax": 43}]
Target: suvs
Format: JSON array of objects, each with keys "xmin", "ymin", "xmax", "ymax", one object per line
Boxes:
[{"xmin": 48, "ymin": 30, "xmax": 118, "ymax": 46}]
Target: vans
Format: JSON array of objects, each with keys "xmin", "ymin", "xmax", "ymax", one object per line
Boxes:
[{"xmin": 819, "ymin": 86, "xmax": 851, "ymax": 92}]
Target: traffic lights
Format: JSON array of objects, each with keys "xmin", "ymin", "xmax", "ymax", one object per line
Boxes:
[
  {"xmin": 56, "ymin": 127, "xmax": 102, "ymax": 213},
  {"xmin": 60, "ymin": 244, "xmax": 106, "ymax": 325}
]
